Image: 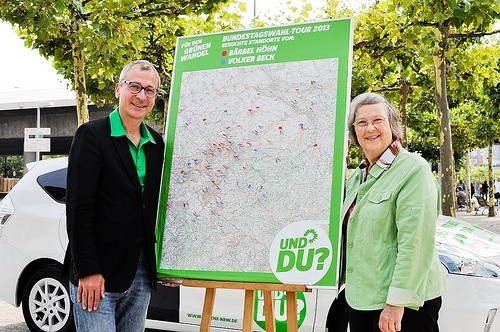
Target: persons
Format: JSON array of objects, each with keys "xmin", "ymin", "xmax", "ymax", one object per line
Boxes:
[
  {"xmin": 63, "ymin": 59, "xmax": 183, "ymax": 332},
  {"xmin": 339, "ymin": 92, "xmax": 446, "ymax": 332},
  {"xmin": 456, "ymin": 176, "xmax": 500, "ymax": 210}
]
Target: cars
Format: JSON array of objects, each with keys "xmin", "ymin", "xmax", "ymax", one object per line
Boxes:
[{"xmin": 1, "ymin": 156, "xmax": 500, "ymax": 331}]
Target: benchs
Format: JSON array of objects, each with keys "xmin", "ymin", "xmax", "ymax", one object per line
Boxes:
[
  {"xmin": 474, "ymin": 195, "xmax": 497, "ymax": 215},
  {"xmin": 455, "ymin": 195, "xmax": 474, "ymax": 213}
]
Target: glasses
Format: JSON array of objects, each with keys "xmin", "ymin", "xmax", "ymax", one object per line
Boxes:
[
  {"xmin": 120, "ymin": 81, "xmax": 158, "ymax": 98},
  {"xmin": 351, "ymin": 116, "xmax": 390, "ymax": 127}
]
